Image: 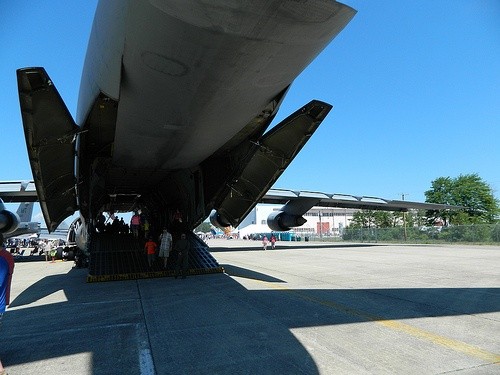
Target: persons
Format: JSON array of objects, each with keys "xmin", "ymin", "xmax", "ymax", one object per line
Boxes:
[
  {"xmin": 0, "ymin": 232, "xmax": 15, "ymax": 375},
  {"xmin": 5, "ymin": 211, "xmax": 276, "ymax": 279}
]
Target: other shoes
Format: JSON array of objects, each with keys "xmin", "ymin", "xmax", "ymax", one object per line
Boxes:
[
  {"xmin": 182, "ymin": 275, "xmax": 186, "ymax": 279},
  {"xmin": 175, "ymin": 275, "xmax": 177, "ymax": 279}
]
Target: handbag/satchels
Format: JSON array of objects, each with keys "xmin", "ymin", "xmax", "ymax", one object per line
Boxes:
[{"xmin": 159, "ymin": 233, "xmax": 163, "ymax": 245}]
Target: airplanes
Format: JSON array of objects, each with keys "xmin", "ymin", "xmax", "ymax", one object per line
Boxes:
[{"xmin": 0, "ymin": 0, "xmax": 471, "ymax": 283}]
